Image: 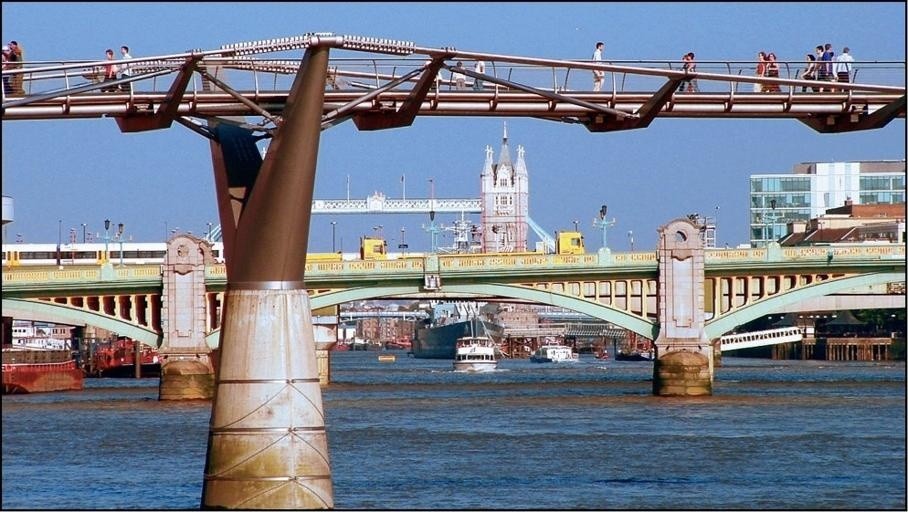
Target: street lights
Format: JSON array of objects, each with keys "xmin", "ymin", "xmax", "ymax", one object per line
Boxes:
[
  {"xmin": 330, "ymin": 220, "xmax": 338, "ymax": 253},
  {"xmin": 428, "ymin": 176, "xmax": 436, "ymax": 199},
  {"xmin": 769, "ymin": 200, "xmax": 777, "ymax": 242},
  {"xmin": 400, "ymin": 173, "xmax": 407, "ymax": 200},
  {"xmin": 345, "ymin": 174, "xmax": 351, "ymax": 200},
  {"xmin": 573, "ymin": 204, "xmax": 636, "ymax": 251},
  {"xmin": 373, "ymin": 223, "xmax": 405, "ymax": 255},
  {"xmin": 81, "ymin": 223, "xmax": 88, "ymax": 243},
  {"xmin": 101, "ymin": 216, "xmax": 125, "ymax": 268}
]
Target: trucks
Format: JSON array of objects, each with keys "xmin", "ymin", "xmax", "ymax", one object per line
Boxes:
[{"xmin": 305, "ymin": 231, "xmax": 585, "ymax": 261}]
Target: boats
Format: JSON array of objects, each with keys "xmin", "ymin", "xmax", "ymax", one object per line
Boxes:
[
  {"xmin": 452, "ymin": 336, "xmax": 499, "ymax": 373},
  {"xmin": 530, "ymin": 345, "xmax": 579, "ymax": 363},
  {"xmin": 408, "ymin": 209, "xmax": 504, "ymax": 358}
]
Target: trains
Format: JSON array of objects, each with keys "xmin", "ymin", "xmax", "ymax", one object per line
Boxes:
[{"xmin": 2, "ymin": 242, "xmax": 225, "ymax": 267}]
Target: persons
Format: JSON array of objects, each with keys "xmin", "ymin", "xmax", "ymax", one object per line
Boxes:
[
  {"xmin": 678, "ymin": 52, "xmax": 700, "ymax": 93},
  {"xmin": 452, "ymin": 61, "xmax": 467, "ymax": 90},
  {"xmin": 2, "ymin": 41, "xmax": 26, "ymax": 99},
  {"xmin": 752, "ymin": 52, "xmax": 767, "ymax": 93},
  {"xmin": 120, "ymin": 46, "xmax": 133, "ymax": 94},
  {"xmin": 765, "ymin": 53, "xmax": 781, "ymax": 93},
  {"xmin": 591, "ymin": 42, "xmax": 604, "ymax": 91},
  {"xmin": 100, "ymin": 49, "xmax": 118, "ymax": 94},
  {"xmin": 800, "ymin": 44, "xmax": 855, "ymax": 94},
  {"xmin": 472, "ymin": 60, "xmax": 485, "ymax": 91}
]
